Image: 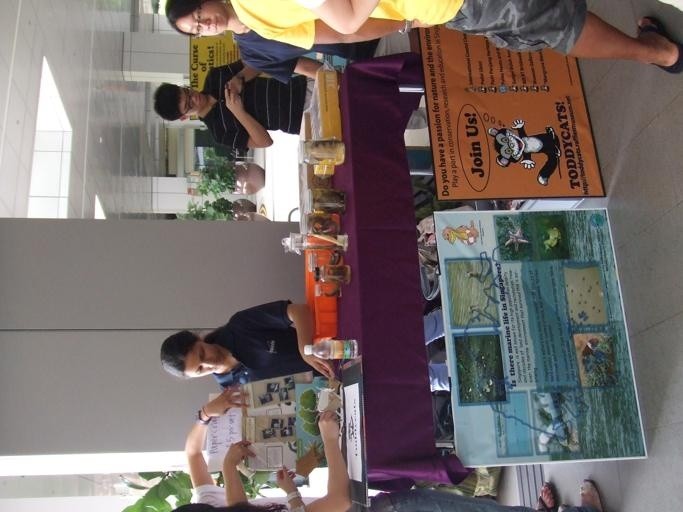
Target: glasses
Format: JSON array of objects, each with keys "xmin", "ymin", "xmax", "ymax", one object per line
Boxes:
[
  {"xmin": 192, "ymin": 5, "xmax": 206, "ymax": 39},
  {"xmin": 182, "ymin": 86, "xmax": 193, "ymax": 116}
]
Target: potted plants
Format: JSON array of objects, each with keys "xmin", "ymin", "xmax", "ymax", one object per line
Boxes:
[{"xmin": 177, "ymin": 161, "xmax": 265, "ymax": 220}]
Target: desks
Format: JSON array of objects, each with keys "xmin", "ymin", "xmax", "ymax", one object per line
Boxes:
[{"xmin": 334, "ymin": 70, "xmax": 457, "ymax": 485}]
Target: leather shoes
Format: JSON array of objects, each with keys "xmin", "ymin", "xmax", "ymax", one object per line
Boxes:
[{"xmin": 579, "ymin": 480, "xmax": 604, "ymax": 512}]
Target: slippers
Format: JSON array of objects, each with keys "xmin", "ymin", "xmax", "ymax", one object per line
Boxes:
[
  {"xmin": 635, "ymin": 12, "xmax": 683, "ymax": 76},
  {"xmin": 535, "ymin": 480, "xmax": 559, "ymax": 510}
]
